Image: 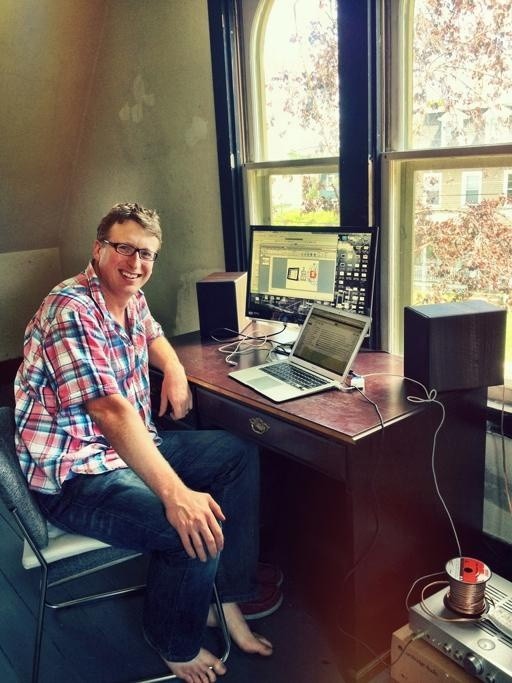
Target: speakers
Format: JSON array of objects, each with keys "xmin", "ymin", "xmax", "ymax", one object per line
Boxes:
[
  {"xmin": 403, "ymin": 299, "xmax": 507, "ymax": 393},
  {"xmin": 196, "ymin": 271, "xmax": 253, "ymax": 343}
]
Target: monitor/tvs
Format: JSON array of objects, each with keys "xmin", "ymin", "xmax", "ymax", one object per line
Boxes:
[{"xmin": 245, "ymin": 225, "xmax": 380, "ymax": 337}]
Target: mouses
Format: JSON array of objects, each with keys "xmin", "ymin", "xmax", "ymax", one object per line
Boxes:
[{"xmin": 346, "ymin": 374, "xmax": 364, "ymax": 387}]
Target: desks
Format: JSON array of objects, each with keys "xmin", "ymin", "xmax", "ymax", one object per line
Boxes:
[{"xmin": 149, "ymin": 322, "xmax": 488, "ymax": 683}]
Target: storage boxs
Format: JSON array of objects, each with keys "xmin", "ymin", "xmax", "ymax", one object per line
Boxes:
[{"xmin": 391, "ymin": 622, "xmax": 483, "ymax": 683}]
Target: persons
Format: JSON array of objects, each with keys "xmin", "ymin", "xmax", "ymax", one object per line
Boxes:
[{"xmin": 13, "ymin": 203, "xmax": 275, "ymax": 682}]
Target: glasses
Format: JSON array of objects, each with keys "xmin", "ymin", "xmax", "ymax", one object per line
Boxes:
[{"xmin": 103, "ymin": 238, "xmax": 158, "ymax": 262}]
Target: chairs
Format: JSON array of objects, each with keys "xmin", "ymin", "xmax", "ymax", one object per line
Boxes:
[{"xmin": 0, "ymin": 407, "xmax": 231, "ymax": 683}]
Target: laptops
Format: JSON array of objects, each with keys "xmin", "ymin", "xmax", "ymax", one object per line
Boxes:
[{"xmin": 227, "ymin": 303, "xmax": 373, "ymax": 404}]
